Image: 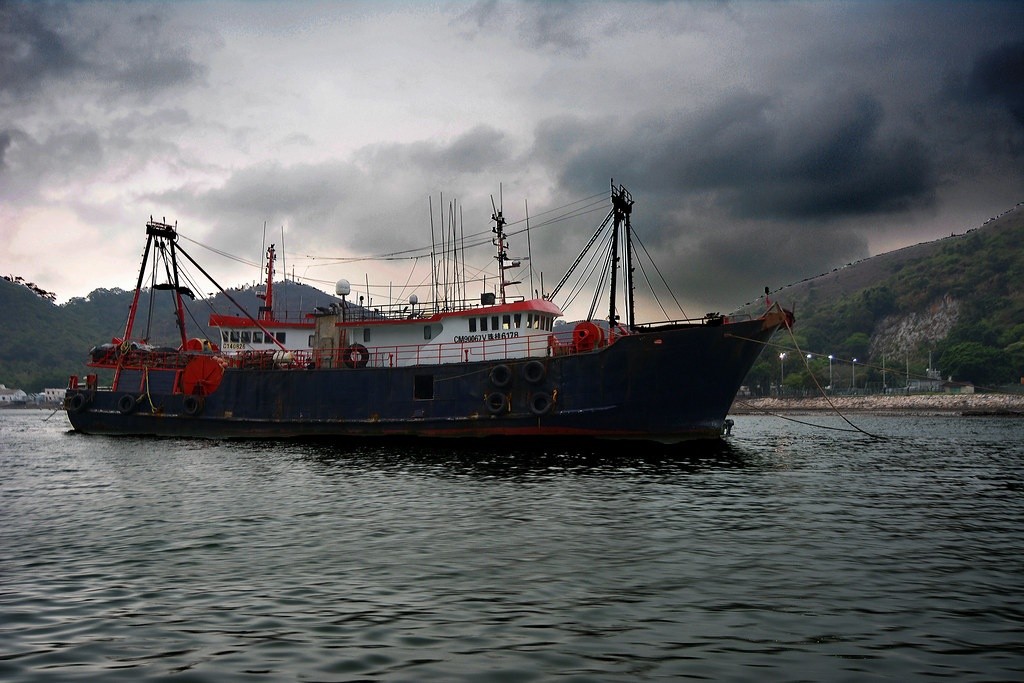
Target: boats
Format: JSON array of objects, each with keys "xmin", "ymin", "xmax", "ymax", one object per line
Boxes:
[{"xmin": 60, "ymin": 176, "xmax": 798, "ymax": 453}]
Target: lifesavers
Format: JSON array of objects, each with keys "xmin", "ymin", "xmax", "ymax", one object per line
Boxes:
[
  {"xmin": 520, "ymin": 361, "xmax": 546, "ymax": 384},
  {"xmin": 483, "ymin": 391, "xmax": 509, "ymax": 414},
  {"xmin": 487, "ymin": 364, "xmax": 514, "ymax": 388},
  {"xmin": 342, "ymin": 343, "xmax": 370, "ymax": 369},
  {"xmin": 181, "ymin": 397, "xmax": 199, "ymax": 415},
  {"xmin": 69, "ymin": 393, "xmax": 86, "ymax": 414},
  {"xmin": 117, "ymin": 394, "xmax": 137, "ymax": 415},
  {"xmin": 526, "ymin": 391, "xmax": 554, "ymax": 418}
]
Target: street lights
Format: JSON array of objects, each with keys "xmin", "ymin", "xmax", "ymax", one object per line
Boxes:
[
  {"xmin": 806, "ymin": 354, "xmax": 812, "ymax": 375},
  {"xmin": 779, "ymin": 352, "xmax": 785, "ymax": 384},
  {"xmin": 828, "ymin": 354, "xmax": 834, "ymax": 386},
  {"xmin": 852, "ymin": 359, "xmax": 858, "ymax": 388}
]
